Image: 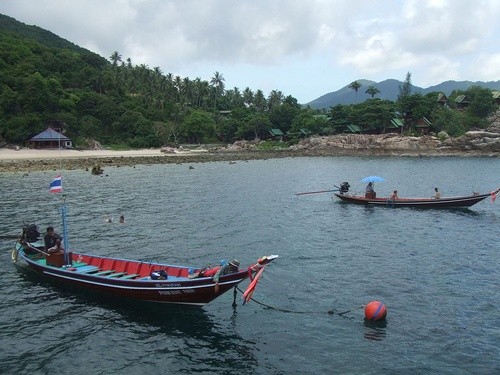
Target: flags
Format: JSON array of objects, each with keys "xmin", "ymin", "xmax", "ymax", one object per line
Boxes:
[{"xmin": 50, "ymin": 176, "xmax": 63, "ymax": 192}]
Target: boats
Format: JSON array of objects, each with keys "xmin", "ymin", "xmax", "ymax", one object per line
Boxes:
[
  {"xmin": 10, "ymin": 228, "xmax": 280, "ymax": 309},
  {"xmin": 333, "ymin": 185, "xmax": 500, "ymax": 210}
]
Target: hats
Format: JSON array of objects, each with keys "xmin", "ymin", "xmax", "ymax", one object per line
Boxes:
[{"xmin": 230, "ymin": 259, "xmax": 240, "ymax": 267}]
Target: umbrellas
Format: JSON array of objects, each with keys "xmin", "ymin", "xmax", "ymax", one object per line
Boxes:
[{"xmin": 360, "ymin": 176, "xmax": 386, "ymax": 194}]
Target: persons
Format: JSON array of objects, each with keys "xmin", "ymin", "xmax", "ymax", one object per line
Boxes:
[
  {"xmin": 365, "ymin": 182, "xmax": 375, "ymax": 195},
  {"xmin": 389, "ymin": 190, "xmax": 399, "ymax": 201},
  {"xmin": 44, "ymin": 227, "xmax": 63, "ymax": 254},
  {"xmin": 431, "ymin": 187, "xmax": 440, "ymax": 199}
]
